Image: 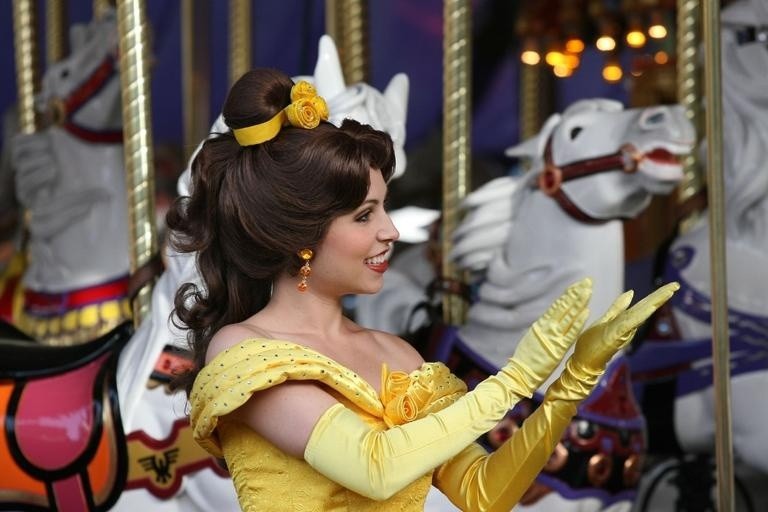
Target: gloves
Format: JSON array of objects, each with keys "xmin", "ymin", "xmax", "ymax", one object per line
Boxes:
[
  {"xmin": 435, "ymin": 280, "xmax": 681, "ymax": 512},
  {"xmin": 303, "ymin": 281, "xmax": 592, "ymax": 502}
]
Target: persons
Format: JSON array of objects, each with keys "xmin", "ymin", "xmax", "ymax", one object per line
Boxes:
[{"xmin": 163, "ymin": 64, "xmax": 685, "ymax": 510}]
